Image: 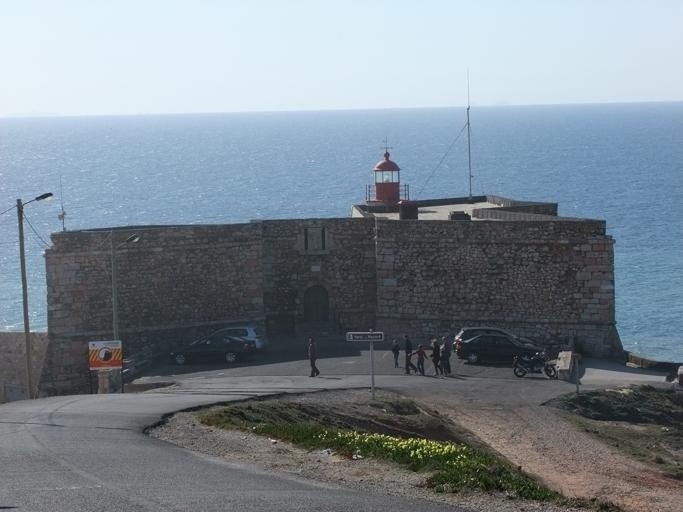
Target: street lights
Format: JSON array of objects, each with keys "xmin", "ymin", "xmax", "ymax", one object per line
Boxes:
[
  {"xmin": 16, "ymin": 192, "xmax": 55, "ymax": 400},
  {"xmin": 109, "ymin": 230, "xmax": 141, "ymax": 341}
]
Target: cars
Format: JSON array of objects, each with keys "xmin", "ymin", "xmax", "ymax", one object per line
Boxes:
[
  {"xmin": 456, "ymin": 333, "xmax": 543, "ymax": 364},
  {"xmin": 188, "ymin": 326, "xmax": 267, "ymax": 349},
  {"xmin": 168, "ymin": 333, "xmax": 256, "ymax": 366}
]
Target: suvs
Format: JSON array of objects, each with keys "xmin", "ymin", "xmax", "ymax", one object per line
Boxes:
[{"xmin": 452, "ymin": 326, "xmax": 538, "ymax": 354}]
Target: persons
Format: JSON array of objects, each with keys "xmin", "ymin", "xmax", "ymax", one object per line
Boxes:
[
  {"xmin": 407, "ymin": 343, "xmax": 429, "ymax": 376},
  {"xmin": 444, "ymin": 335, "xmax": 451, "ymax": 373},
  {"xmin": 439, "ymin": 337, "xmax": 448, "ymax": 379},
  {"xmin": 402, "ymin": 335, "xmax": 417, "ymax": 375},
  {"xmin": 391, "ymin": 338, "xmax": 401, "ymax": 369},
  {"xmin": 427, "ymin": 338, "xmax": 443, "ymax": 377},
  {"xmin": 307, "ymin": 337, "xmax": 320, "ymax": 378}
]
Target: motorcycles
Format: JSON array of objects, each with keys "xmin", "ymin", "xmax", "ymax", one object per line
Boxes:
[{"xmin": 512, "ymin": 349, "xmax": 556, "ymax": 378}]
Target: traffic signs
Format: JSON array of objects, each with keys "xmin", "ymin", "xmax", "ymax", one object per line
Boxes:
[{"xmin": 345, "ymin": 331, "xmax": 385, "ymax": 343}]
